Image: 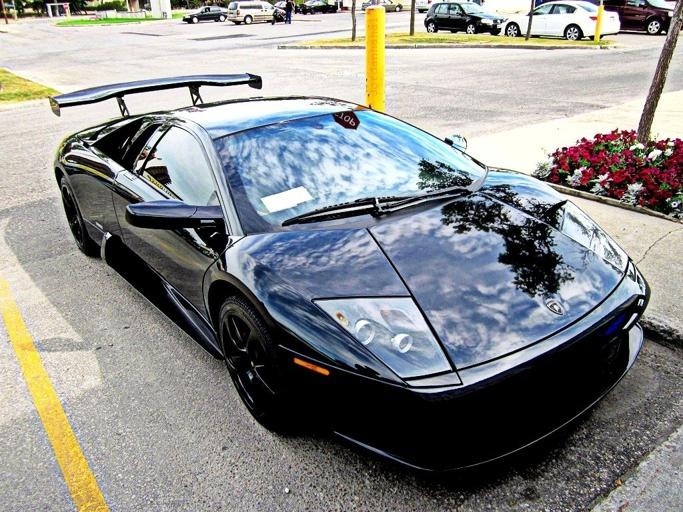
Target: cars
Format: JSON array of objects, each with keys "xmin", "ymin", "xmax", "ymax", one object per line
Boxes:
[
  {"xmin": 275, "ymin": 0, "xmax": 338, "ymax": 15},
  {"xmin": 415, "ymin": 0, "xmax": 675, "ymax": 42},
  {"xmin": 362, "ymin": 0, "xmax": 403, "ymax": 12},
  {"xmin": 182, "ymin": 6, "xmax": 227, "ymax": 24},
  {"xmin": 48, "ymin": 72, "xmax": 652, "ymax": 483}
]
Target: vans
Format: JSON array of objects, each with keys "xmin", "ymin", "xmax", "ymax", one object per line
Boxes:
[{"xmin": 227, "ymin": 1, "xmax": 288, "ymax": 25}]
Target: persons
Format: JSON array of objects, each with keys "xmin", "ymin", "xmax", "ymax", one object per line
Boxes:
[{"xmin": 284, "ymin": 0, "xmax": 292, "ymax": 24}]
